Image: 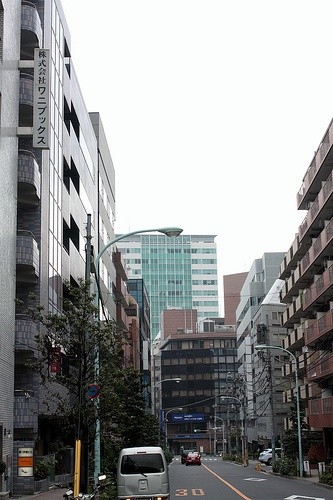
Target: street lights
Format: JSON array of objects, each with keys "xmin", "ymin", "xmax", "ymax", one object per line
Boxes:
[
  {"xmin": 221, "ymin": 395, "xmax": 243, "ymax": 464},
  {"xmin": 96, "ymin": 228, "xmax": 184, "ymax": 500},
  {"xmin": 153, "ymin": 377, "xmax": 181, "ymax": 387},
  {"xmin": 166, "ymin": 407, "xmax": 181, "ymax": 443},
  {"xmin": 255, "ymin": 345, "xmax": 303, "ymax": 478},
  {"xmin": 214, "ymin": 416, "xmax": 225, "ymax": 454}
]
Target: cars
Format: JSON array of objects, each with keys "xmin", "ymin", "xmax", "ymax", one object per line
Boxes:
[
  {"xmin": 181, "ymin": 450, "xmax": 194, "ymax": 464},
  {"xmin": 186, "ymin": 452, "xmax": 201, "ymax": 465}
]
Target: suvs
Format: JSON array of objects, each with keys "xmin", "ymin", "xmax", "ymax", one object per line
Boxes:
[{"xmin": 259, "ymin": 448, "xmax": 284, "ymax": 466}]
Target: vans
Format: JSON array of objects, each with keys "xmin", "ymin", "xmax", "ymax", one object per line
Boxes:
[{"xmin": 117, "ymin": 446, "xmax": 169, "ymax": 500}]
